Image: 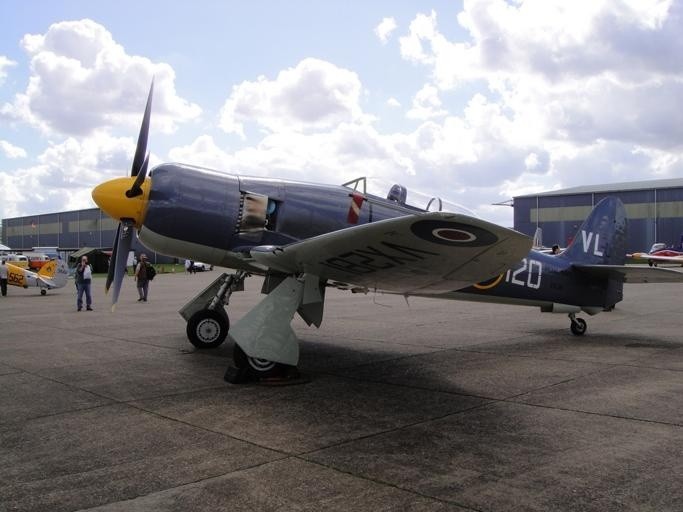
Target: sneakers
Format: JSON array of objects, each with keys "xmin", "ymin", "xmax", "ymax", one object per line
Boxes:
[
  {"xmin": 138, "ymin": 298, "xmax": 147, "ymax": 302},
  {"xmin": 77, "ymin": 306, "xmax": 92, "ymax": 311}
]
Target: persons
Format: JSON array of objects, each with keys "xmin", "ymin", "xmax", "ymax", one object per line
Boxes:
[
  {"xmin": 133, "ymin": 256, "xmax": 138, "ymax": 273},
  {"xmin": 134, "ymin": 253, "xmax": 151, "ymax": 302},
  {"xmin": 74, "ymin": 256, "xmax": 93, "ymax": 310},
  {"xmin": 189, "ymin": 260, "xmax": 194, "ymax": 274},
  {"xmin": 550, "ymin": 244, "xmax": 561, "ymax": 255},
  {"xmin": 185, "ymin": 259, "xmax": 191, "ymax": 275},
  {"xmin": 0, "ymin": 259, "xmax": 9, "ymax": 296},
  {"xmin": 58, "ymin": 255, "xmax": 62, "ymax": 267}
]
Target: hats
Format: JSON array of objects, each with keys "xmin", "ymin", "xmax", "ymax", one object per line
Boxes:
[{"xmin": 140, "ymin": 253, "xmax": 147, "ymax": 259}]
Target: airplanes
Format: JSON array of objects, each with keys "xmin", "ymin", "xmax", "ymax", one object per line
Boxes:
[
  {"xmin": 632, "ymin": 243, "xmax": 683, "ymax": 267},
  {"xmin": 0, "ymin": 259, "xmax": 70, "ymax": 294},
  {"xmin": 92, "ymin": 76, "xmax": 628, "ymax": 379}
]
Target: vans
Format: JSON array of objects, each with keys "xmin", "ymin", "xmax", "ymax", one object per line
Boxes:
[{"xmin": 0, "ymin": 255, "xmax": 49, "ymax": 270}]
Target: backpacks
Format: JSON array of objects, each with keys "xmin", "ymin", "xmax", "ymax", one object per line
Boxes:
[{"xmin": 144, "ymin": 262, "xmax": 156, "ymax": 281}]
